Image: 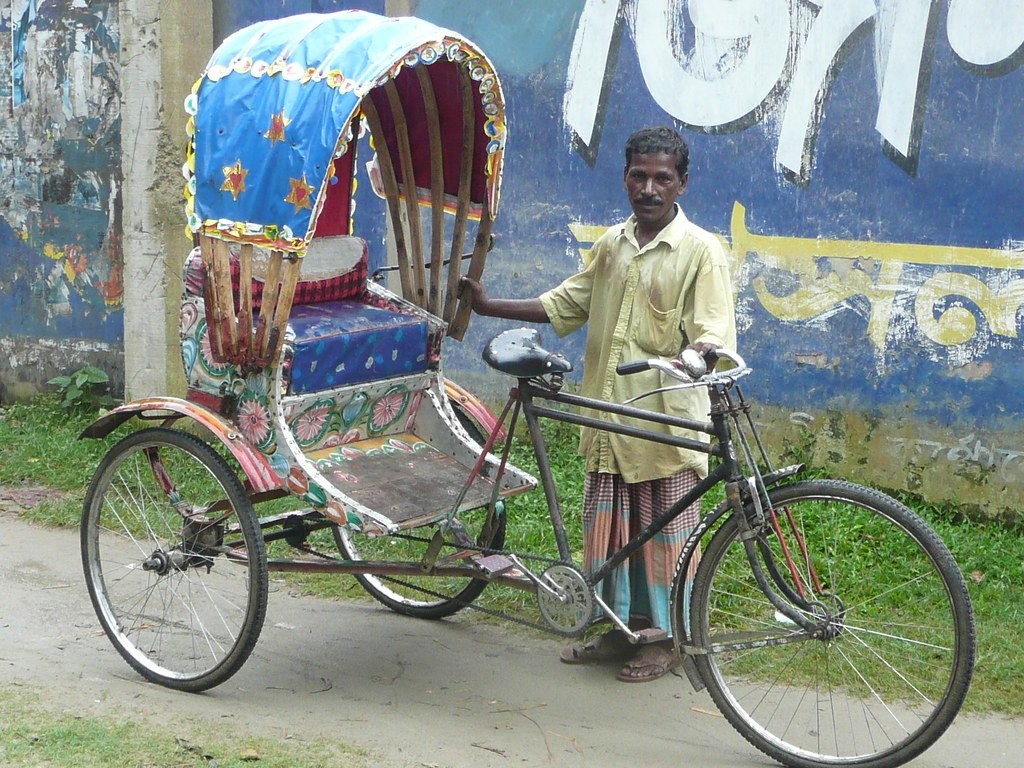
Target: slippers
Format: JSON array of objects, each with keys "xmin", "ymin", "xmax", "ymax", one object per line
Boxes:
[
  {"xmin": 615, "ymin": 645, "xmax": 681, "ymax": 682},
  {"xmin": 559, "ymin": 632, "xmax": 627, "ymax": 662}
]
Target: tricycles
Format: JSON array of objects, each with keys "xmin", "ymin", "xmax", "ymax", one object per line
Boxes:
[{"xmin": 69, "ymin": 8, "xmax": 976, "ymax": 768}]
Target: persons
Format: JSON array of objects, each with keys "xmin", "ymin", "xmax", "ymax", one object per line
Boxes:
[{"xmin": 458, "ymin": 127, "xmax": 738, "ymax": 684}]
